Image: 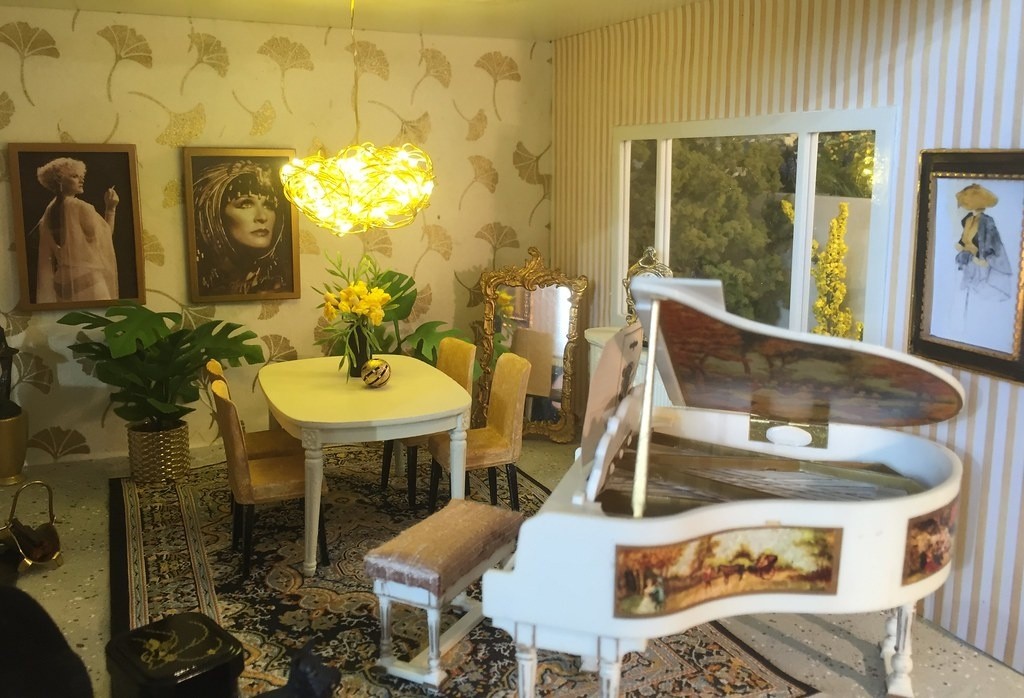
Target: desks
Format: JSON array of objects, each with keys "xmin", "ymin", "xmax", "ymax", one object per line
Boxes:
[{"xmin": 259, "ymin": 353, "xmax": 472, "ymax": 578}]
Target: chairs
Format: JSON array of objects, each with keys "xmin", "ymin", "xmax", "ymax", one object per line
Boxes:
[
  {"xmin": 426, "ymin": 352, "xmax": 531, "ymax": 511},
  {"xmin": 210, "ymin": 379, "xmax": 332, "ymax": 572},
  {"xmin": 381, "ymin": 336, "xmax": 477, "ymax": 505},
  {"xmin": 206, "ymin": 358, "xmax": 306, "ymax": 544}
]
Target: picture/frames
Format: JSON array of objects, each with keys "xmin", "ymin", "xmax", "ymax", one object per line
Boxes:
[
  {"xmin": 906, "ymin": 148, "xmax": 1024, "ymax": 385},
  {"xmin": 7, "ymin": 142, "xmax": 147, "ymax": 309},
  {"xmin": 182, "ymin": 146, "xmax": 302, "ymax": 305}
]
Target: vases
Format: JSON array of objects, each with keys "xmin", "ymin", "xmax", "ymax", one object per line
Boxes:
[
  {"xmin": 0, "ymin": 410, "xmax": 29, "ymax": 487},
  {"xmin": 349, "ymin": 324, "xmax": 372, "ymax": 377}
]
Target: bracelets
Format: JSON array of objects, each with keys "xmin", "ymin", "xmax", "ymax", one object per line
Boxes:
[{"xmin": 104, "ymin": 211, "xmax": 116, "ymax": 215}]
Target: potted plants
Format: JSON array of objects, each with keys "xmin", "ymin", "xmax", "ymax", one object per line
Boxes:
[{"xmin": 56, "ymin": 299, "xmax": 265, "ymax": 484}]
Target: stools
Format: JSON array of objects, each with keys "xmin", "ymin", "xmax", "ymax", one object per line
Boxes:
[{"xmin": 365, "ymin": 499, "xmax": 526, "ymax": 692}]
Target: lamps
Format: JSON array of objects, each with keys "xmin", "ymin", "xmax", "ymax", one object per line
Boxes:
[{"xmin": 280, "ymin": 1, "xmax": 434, "ymax": 238}]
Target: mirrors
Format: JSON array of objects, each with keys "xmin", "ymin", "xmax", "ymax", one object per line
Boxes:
[{"xmin": 471, "ymin": 247, "xmax": 589, "ymax": 445}]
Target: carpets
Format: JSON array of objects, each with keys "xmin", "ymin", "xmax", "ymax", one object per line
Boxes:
[{"xmin": 111, "ymin": 443, "xmax": 821, "ymax": 698}]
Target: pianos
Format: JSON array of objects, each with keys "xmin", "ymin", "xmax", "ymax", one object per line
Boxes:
[{"xmin": 480, "ymin": 276, "xmax": 968, "ymax": 698}]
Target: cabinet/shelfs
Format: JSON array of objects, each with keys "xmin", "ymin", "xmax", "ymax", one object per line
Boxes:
[{"xmin": 583, "ymin": 325, "xmax": 672, "ymax": 407}]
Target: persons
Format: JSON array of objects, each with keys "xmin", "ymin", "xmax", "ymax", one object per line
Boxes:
[
  {"xmin": 198, "ymin": 160, "xmax": 294, "ymax": 294},
  {"xmin": 28, "ymin": 157, "xmax": 120, "ymax": 304}
]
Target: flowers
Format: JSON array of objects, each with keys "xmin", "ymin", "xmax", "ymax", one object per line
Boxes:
[{"xmin": 308, "ymin": 249, "xmax": 403, "ymax": 382}]
